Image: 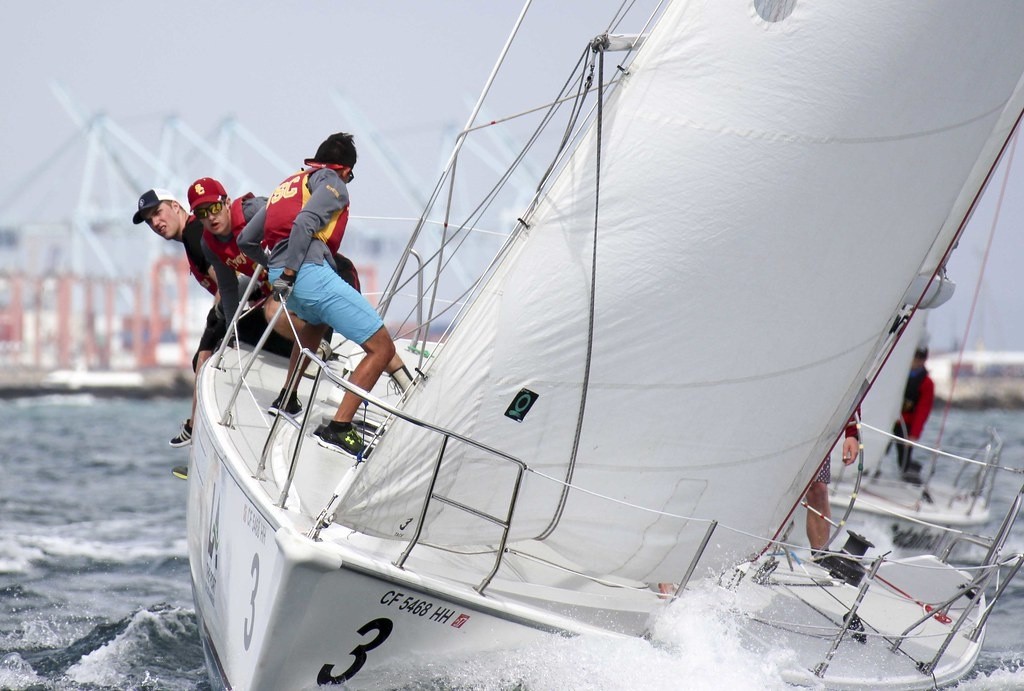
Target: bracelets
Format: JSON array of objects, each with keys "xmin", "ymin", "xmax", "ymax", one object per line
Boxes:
[{"xmin": 280, "ymin": 272, "xmax": 296, "ymax": 284}]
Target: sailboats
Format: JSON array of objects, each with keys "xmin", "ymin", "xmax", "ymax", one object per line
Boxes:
[
  {"xmin": 778, "ymin": 290, "xmax": 1004, "ymax": 551},
  {"xmin": 181, "ymin": 0, "xmax": 1024, "ymax": 691}
]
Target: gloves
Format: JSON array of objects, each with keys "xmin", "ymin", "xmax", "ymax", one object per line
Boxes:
[
  {"xmin": 228, "ymin": 329, "xmax": 240, "ymax": 348},
  {"xmin": 273, "ymin": 272, "xmax": 295, "ymax": 302}
]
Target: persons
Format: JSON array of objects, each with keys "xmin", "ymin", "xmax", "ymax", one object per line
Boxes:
[
  {"xmin": 236, "ymin": 132, "xmax": 396, "ymax": 462},
  {"xmin": 133, "ymin": 176, "xmax": 416, "ymax": 482},
  {"xmin": 656, "ymin": 345, "xmax": 934, "ymax": 601}
]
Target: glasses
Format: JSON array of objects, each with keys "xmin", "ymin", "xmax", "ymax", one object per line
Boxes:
[
  {"xmin": 194, "ymin": 199, "xmax": 227, "ymax": 219},
  {"xmin": 349, "ymin": 170, "xmax": 355, "ymax": 184}
]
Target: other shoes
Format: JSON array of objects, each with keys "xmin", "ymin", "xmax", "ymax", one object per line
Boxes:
[{"xmin": 171, "ymin": 465, "xmax": 188, "ymax": 480}]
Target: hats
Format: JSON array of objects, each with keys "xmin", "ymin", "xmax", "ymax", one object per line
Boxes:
[
  {"xmin": 187, "ymin": 178, "xmax": 227, "ymax": 212},
  {"xmin": 132, "ymin": 189, "xmax": 176, "ymax": 224}
]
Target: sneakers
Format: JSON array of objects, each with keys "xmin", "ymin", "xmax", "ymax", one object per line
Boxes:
[
  {"xmin": 268, "ymin": 393, "xmax": 304, "ymax": 418},
  {"xmin": 318, "ymin": 422, "xmax": 373, "ymax": 462},
  {"xmin": 169, "ymin": 417, "xmax": 193, "ymax": 447}
]
